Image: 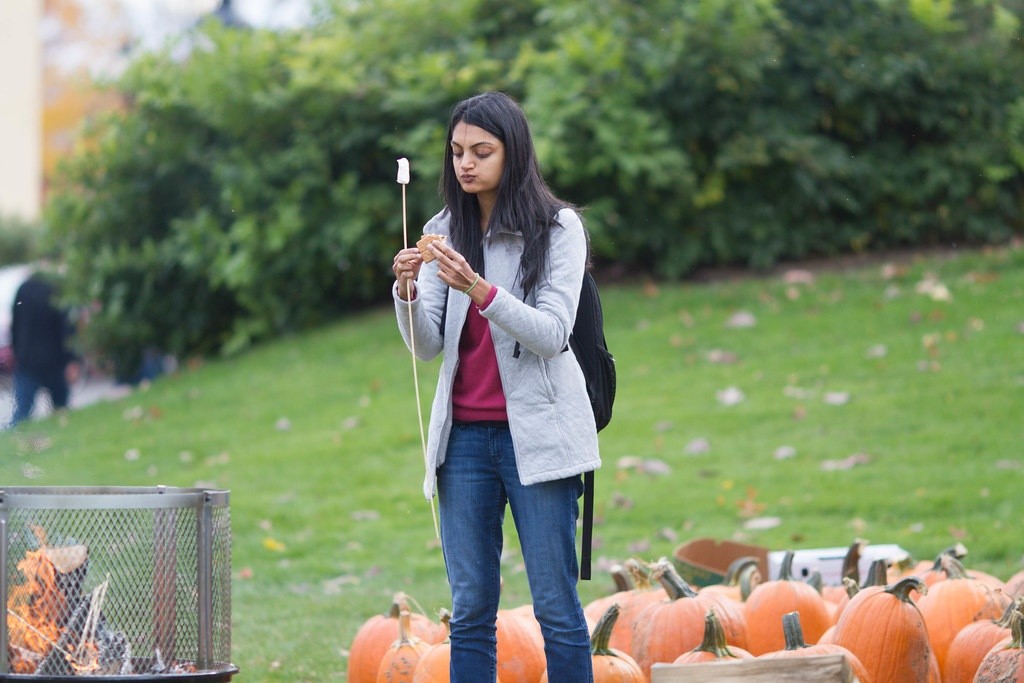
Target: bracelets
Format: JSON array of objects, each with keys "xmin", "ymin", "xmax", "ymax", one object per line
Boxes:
[{"xmin": 463, "ymin": 273, "xmax": 480, "ymax": 294}]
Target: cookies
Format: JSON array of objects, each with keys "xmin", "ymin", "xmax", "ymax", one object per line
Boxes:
[{"xmin": 416, "ymin": 235, "xmax": 445, "ymax": 263}]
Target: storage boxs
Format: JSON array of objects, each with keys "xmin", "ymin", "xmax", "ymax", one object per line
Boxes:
[
  {"xmin": 649, "ymin": 654, "xmax": 860, "ymax": 683},
  {"xmin": 767, "ymin": 543, "xmax": 910, "ymax": 585},
  {"xmin": 671, "ymin": 539, "xmax": 771, "ymax": 588}
]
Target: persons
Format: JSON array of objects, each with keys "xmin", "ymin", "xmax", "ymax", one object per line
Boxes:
[
  {"xmin": 6, "ymin": 270, "xmax": 75, "ymax": 426},
  {"xmin": 391, "ymin": 91, "xmax": 603, "ymax": 683}
]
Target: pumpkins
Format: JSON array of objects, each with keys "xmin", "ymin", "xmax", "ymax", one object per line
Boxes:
[{"xmin": 348, "ymin": 538, "xmax": 1023, "ymax": 683}]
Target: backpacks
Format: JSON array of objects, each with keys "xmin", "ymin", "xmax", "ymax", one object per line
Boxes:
[{"xmin": 513, "ymin": 205, "xmax": 616, "ymax": 580}]
